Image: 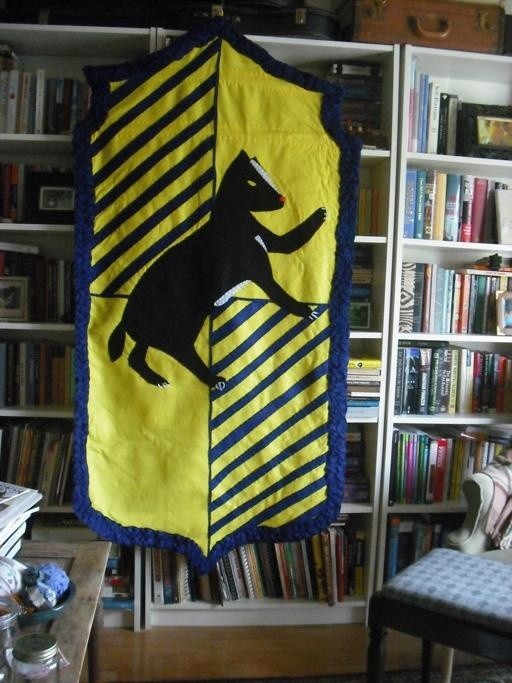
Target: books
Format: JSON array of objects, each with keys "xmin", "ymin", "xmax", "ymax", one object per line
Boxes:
[
  {"xmin": 0, "ymin": 341, "xmax": 74, "ymax": 412},
  {"xmin": 0, "ymin": 242, "xmax": 75, "ymax": 324},
  {"xmin": 151, "ymin": 510, "xmax": 459, "ymax": 606},
  {"xmin": 0, "ymin": 45, "xmax": 93, "ymax": 135},
  {"xmin": 1, "ymin": 161, "xmax": 78, "ymax": 225},
  {"xmin": 31, "ymin": 513, "xmax": 134, "ymax": 613},
  {"xmin": 330, "ymin": 59, "xmax": 392, "ymax": 507},
  {"xmin": 0, "ymin": 479, "xmax": 43, "ymax": 558},
  {"xmin": 0, "ymin": 417, "xmax": 75, "ymax": 507},
  {"xmin": 389, "ymin": 58, "xmax": 512, "ymax": 508}
]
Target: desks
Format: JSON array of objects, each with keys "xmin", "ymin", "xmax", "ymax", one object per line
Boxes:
[{"xmin": 0, "ymin": 540, "xmax": 114, "ymax": 683}]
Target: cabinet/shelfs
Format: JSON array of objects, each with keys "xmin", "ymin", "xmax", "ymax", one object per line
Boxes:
[
  {"xmin": 376, "ymin": 45, "xmax": 512, "ymax": 595},
  {"xmin": 1, "ymin": 21, "xmax": 401, "ymax": 633}
]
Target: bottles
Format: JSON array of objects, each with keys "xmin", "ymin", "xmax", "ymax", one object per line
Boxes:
[{"xmin": 0, "ymin": 597, "xmax": 62, "ymax": 683}]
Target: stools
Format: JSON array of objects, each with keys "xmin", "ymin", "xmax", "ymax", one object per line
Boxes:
[{"xmin": 368, "ymin": 548, "xmax": 512, "ymax": 682}]
